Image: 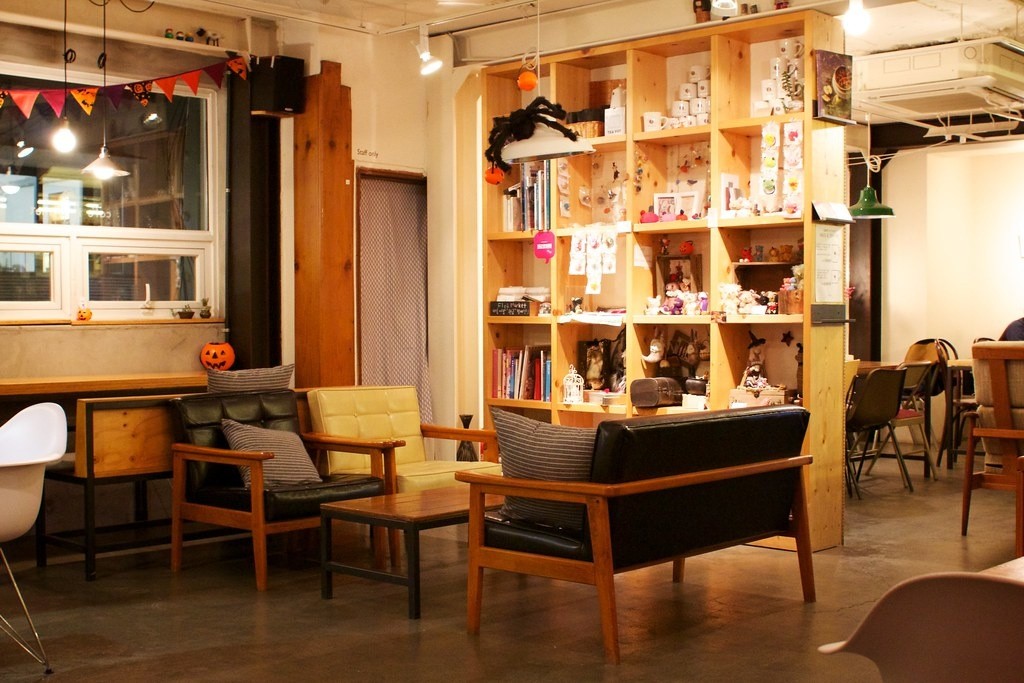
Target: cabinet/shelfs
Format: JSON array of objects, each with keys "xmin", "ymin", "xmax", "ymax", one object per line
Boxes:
[{"xmin": 481, "ymin": 11, "xmax": 848, "ymax": 553}]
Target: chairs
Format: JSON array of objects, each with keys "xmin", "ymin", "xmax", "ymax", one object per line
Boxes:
[
  {"xmin": 299, "ymin": 386, "xmax": 498, "ymax": 570},
  {"xmin": 169, "ymin": 388, "xmax": 395, "ymax": 592},
  {"xmin": 0, "ymin": 402, "xmax": 68, "ymax": 676},
  {"xmin": 843, "ymin": 338, "xmax": 1024, "ymax": 559}
]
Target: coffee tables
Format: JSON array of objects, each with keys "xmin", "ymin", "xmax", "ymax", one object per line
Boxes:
[{"xmin": 319, "ymin": 484, "xmax": 503, "ymax": 619}]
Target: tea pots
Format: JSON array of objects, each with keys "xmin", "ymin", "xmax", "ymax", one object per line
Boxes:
[{"xmin": 610, "ymin": 84, "xmax": 627, "ymax": 108}]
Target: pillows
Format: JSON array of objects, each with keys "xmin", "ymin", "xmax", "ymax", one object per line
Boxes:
[
  {"xmin": 207, "ymin": 364, "xmax": 295, "ymax": 394},
  {"xmin": 221, "ymin": 418, "xmax": 323, "ymax": 490},
  {"xmin": 488, "ymin": 408, "xmax": 599, "ymax": 534}
]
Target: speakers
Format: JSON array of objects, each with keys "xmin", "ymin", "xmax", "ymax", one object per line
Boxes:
[{"xmin": 252, "ymin": 55, "xmax": 304, "ymax": 113}]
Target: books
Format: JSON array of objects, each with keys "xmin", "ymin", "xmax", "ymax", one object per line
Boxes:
[
  {"xmin": 492, "ymin": 345, "xmax": 551, "ymax": 402},
  {"xmin": 502, "ymin": 159, "xmax": 550, "ymax": 232}
]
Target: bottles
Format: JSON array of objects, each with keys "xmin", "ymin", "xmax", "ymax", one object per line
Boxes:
[
  {"xmin": 777, "ymin": 278, "xmax": 804, "ymax": 315},
  {"xmin": 459, "ymin": 413, "xmax": 478, "ymax": 461}
]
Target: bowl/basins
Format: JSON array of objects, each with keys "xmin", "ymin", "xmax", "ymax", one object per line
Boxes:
[{"xmin": 686, "ymin": 378, "xmax": 708, "ymax": 395}]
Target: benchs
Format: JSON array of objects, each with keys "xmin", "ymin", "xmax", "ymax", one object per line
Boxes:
[
  {"xmin": 35, "ymin": 391, "xmax": 207, "ymax": 583},
  {"xmin": 455, "ymin": 403, "xmax": 813, "ymax": 666}
]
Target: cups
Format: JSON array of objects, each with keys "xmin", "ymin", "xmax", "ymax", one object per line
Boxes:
[
  {"xmin": 751, "ymin": 37, "xmax": 804, "ymax": 116},
  {"xmin": 644, "ymin": 65, "xmax": 711, "ymax": 132},
  {"xmin": 844, "ymin": 355, "xmax": 854, "ymax": 361},
  {"xmin": 590, "ymin": 391, "xmax": 607, "ymax": 404}
]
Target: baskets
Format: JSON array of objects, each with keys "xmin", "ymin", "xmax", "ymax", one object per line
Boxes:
[{"xmin": 563, "ymin": 121, "xmax": 604, "ymax": 138}]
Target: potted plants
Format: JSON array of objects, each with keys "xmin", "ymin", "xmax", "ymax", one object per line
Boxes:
[
  {"xmin": 178, "ymin": 305, "xmax": 195, "ymax": 318},
  {"xmin": 200, "ymin": 298, "xmax": 211, "ymax": 318}
]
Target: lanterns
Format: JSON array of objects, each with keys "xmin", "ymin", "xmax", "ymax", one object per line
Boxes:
[
  {"xmin": 77, "ymin": 308, "xmax": 91, "ymax": 320},
  {"xmin": 201, "ymin": 342, "xmax": 235, "ymax": 370}
]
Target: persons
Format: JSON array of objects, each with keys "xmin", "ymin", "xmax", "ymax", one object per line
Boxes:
[{"xmin": 661, "ymin": 280, "xmax": 682, "ymax": 315}]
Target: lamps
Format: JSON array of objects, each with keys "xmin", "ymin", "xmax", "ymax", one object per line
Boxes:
[
  {"xmin": 411, "ymin": 23, "xmax": 443, "ymax": 75},
  {"xmin": 484, "ymin": 0, "xmax": 597, "ymax": 165},
  {"xmin": 13, "ymin": 135, "xmax": 34, "ymax": 158},
  {"xmin": 80, "ymin": 0, "xmax": 131, "ymax": 180},
  {"xmin": 849, "ymin": 114, "xmax": 893, "ymax": 219}
]
